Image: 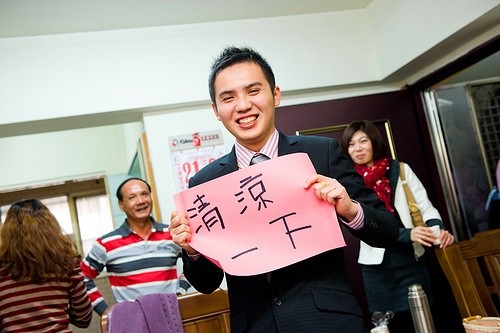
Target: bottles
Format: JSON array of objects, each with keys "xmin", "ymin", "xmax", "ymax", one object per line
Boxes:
[{"xmin": 407, "ymin": 284, "xmax": 437, "ymax": 333}]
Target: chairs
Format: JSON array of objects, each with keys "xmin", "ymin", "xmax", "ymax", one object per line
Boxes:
[
  {"xmin": 100, "ymin": 287, "xmax": 231, "ymax": 333},
  {"xmin": 435, "ymin": 229, "xmax": 500, "ymax": 320}
]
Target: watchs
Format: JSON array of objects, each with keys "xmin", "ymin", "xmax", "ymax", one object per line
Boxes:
[{"xmin": 177, "ymin": 287, "xmax": 186, "ymax": 297}]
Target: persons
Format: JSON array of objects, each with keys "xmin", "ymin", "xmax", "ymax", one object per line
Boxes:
[
  {"xmin": 1, "ymin": 199, "xmax": 93, "ymax": 333},
  {"xmin": 167, "ymin": 43, "xmax": 400, "ymax": 333},
  {"xmin": 337, "ymin": 121, "xmax": 467, "ymax": 333},
  {"xmin": 79, "ymin": 178, "xmax": 193, "ymax": 318}
]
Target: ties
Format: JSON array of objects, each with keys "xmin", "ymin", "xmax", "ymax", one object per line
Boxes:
[{"xmin": 251, "ymin": 154, "xmax": 270, "ymax": 165}]
